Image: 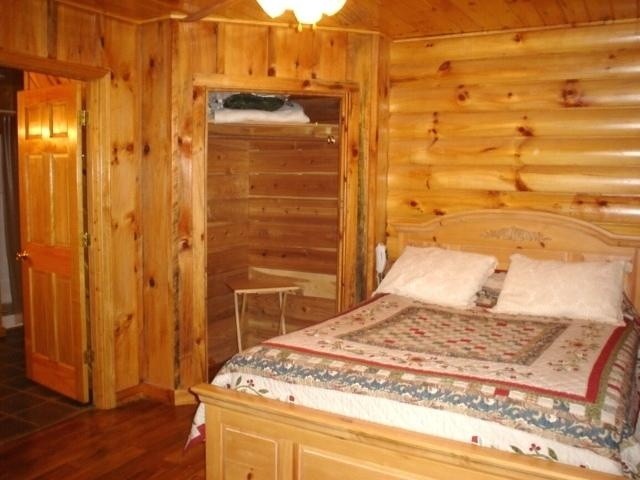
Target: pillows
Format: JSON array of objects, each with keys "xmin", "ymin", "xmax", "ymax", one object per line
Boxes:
[
  {"xmin": 371, "ymin": 244, "xmax": 499, "ymax": 310},
  {"xmin": 485, "ymin": 252, "xmax": 631, "ymax": 328}
]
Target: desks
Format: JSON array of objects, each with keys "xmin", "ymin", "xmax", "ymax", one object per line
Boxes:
[{"xmin": 224, "ymin": 278, "xmax": 301, "ymax": 355}]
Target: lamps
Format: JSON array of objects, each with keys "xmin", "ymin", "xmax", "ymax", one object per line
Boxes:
[{"xmin": 256, "ymin": 0, "xmax": 348, "ymax": 32}]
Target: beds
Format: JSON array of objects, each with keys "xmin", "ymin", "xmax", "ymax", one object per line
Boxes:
[{"xmin": 188, "ymin": 208, "xmax": 640, "ymax": 480}]
